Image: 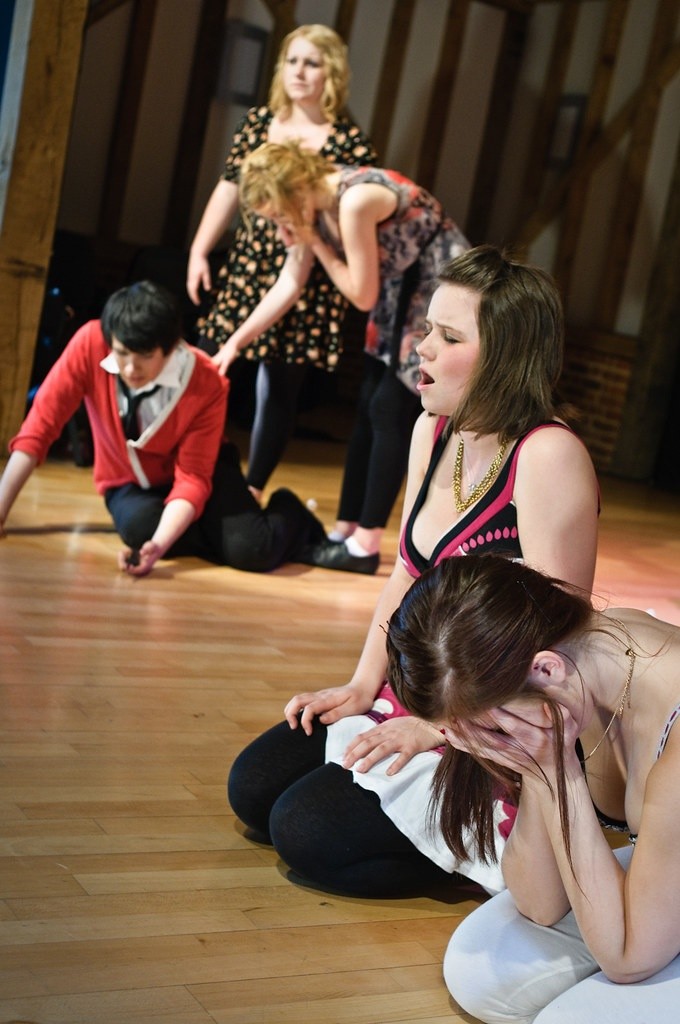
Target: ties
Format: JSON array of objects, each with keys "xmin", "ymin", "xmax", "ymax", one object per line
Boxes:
[{"xmin": 117, "ymin": 374, "xmax": 162, "ymax": 441}]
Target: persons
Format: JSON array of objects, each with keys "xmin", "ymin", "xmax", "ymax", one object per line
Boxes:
[
  {"xmin": 213, "ymin": 139, "xmax": 475, "ymax": 577},
  {"xmin": 0, "ymin": 277, "xmax": 329, "ymax": 578},
  {"xmin": 226, "ymin": 242, "xmax": 605, "ymax": 890},
  {"xmin": 187, "ymin": 21, "xmax": 377, "ymax": 504},
  {"xmin": 388, "ymin": 553, "xmax": 680, "ymax": 1024}
]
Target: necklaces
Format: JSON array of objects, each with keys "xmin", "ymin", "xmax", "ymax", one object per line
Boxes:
[
  {"xmin": 577, "ymin": 613, "xmax": 635, "ymax": 763},
  {"xmin": 451, "ymin": 428, "xmax": 511, "ymax": 512}
]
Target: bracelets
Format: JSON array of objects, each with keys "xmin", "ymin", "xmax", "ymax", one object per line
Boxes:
[{"xmin": 224, "ymin": 342, "xmax": 241, "ymax": 358}]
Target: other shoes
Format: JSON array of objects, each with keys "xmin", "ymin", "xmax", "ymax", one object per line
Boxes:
[
  {"xmin": 313, "ymin": 542, "xmax": 380, "ymax": 575},
  {"xmin": 316, "ymin": 538, "xmax": 343, "ymax": 547}
]
